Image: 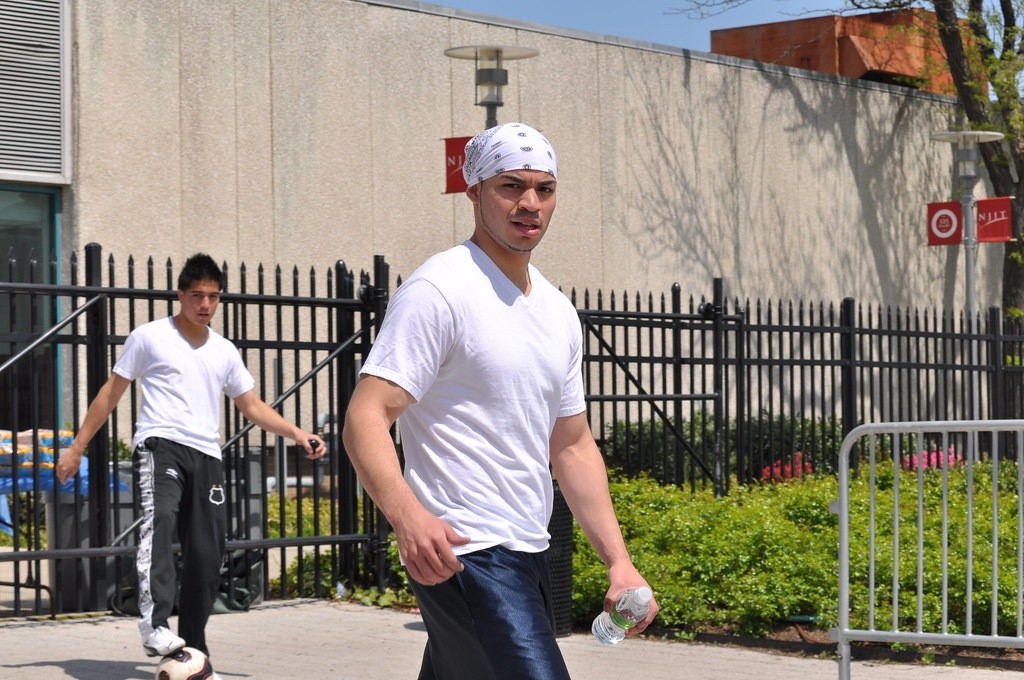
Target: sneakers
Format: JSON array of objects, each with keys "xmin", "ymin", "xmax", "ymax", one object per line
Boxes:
[
  {"xmin": 206, "ymin": 670, "xmax": 222, "ymax": 680},
  {"xmin": 143, "ymin": 626, "xmax": 185, "ymax": 657}
]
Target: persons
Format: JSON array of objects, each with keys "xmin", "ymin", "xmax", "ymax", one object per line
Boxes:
[
  {"xmin": 57, "ymin": 252, "xmax": 328, "ymax": 680},
  {"xmin": 342, "ymin": 121, "xmax": 659, "ymax": 680}
]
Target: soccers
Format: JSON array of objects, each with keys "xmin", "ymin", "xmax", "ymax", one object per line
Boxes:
[{"xmin": 155, "ymin": 646, "xmax": 214, "ymax": 680}]
